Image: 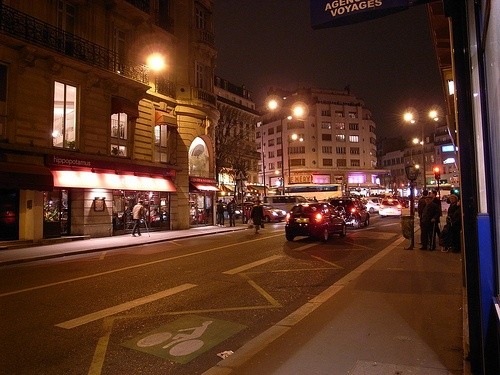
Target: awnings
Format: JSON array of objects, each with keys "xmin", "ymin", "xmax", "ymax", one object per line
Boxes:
[
  {"xmin": 47, "ymin": 164, "xmax": 177, "ymax": 193},
  {"xmin": 191, "ymin": 182, "xmax": 220, "ymax": 191}
]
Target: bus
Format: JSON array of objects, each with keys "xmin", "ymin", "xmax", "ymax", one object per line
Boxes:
[
  {"xmin": 422, "ymin": 183, "xmax": 452, "ymax": 201},
  {"xmin": 281, "ymin": 183, "xmax": 343, "ymax": 202}
]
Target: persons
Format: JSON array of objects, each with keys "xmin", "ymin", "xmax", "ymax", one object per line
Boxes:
[
  {"xmin": 227, "ymin": 200, "xmax": 236, "ymax": 227},
  {"xmin": 250, "ymin": 200, "xmax": 264, "ymax": 234},
  {"xmin": 217, "ymin": 200, "xmax": 226, "ymax": 227},
  {"xmin": 418, "ymin": 190, "xmax": 428, "ymax": 243},
  {"xmin": 131, "ymin": 201, "xmax": 146, "ymax": 237},
  {"xmin": 431, "ymin": 191, "xmax": 442, "ymax": 237},
  {"xmin": 419, "ymin": 196, "xmax": 438, "ymax": 250},
  {"xmin": 440, "ymin": 190, "xmax": 461, "ymax": 253}
]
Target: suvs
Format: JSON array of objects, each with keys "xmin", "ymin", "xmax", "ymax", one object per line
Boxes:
[
  {"xmin": 328, "ymin": 196, "xmax": 372, "ymax": 230},
  {"xmin": 283, "ymin": 202, "xmax": 347, "ymax": 243},
  {"xmin": 378, "ymin": 198, "xmax": 410, "ymax": 218}
]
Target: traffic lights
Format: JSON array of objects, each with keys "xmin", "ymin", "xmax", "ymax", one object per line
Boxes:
[{"xmin": 434, "ymin": 165, "xmax": 441, "ymax": 180}]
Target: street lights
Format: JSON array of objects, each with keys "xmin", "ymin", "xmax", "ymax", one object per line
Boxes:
[
  {"xmin": 265, "ymin": 95, "xmax": 307, "ymax": 195},
  {"xmin": 404, "ymin": 164, "xmax": 419, "ymax": 251},
  {"xmin": 451, "ymin": 183, "xmax": 454, "ymax": 190},
  {"xmin": 401, "ymin": 105, "xmax": 440, "ymax": 195},
  {"xmin": 287, "ymin": 133, "xmax": 304, "ymax": 183}
]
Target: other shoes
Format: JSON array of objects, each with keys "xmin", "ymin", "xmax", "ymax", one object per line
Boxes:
[
  {"xmin": 256, "ymin": 232, "xmax": 259, "ymax": 234},
  {"xmin": 419, "ymin": 247, "xmax": 428, "ymax": 250},
  {"xmin": 132, "ymin": 234, "xmax": 136, "ymax": 237},
  {"xmin": 138, "ymin": 234, "xmax": 140, "ymax": 236}
]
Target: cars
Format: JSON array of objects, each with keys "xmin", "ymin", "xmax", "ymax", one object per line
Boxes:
[
  {"xmin": 242, "ymin": 202, "xmax": 287, "ymax": 224},
  {"xmin": 364, "ymin": 196, "xmax": 382, "ymax": 213},
  {"xmin": 215, "ymin": 201, "xmax": 256, "ymax": 221}
]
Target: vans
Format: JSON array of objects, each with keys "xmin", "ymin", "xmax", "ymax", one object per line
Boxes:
[{"xmin": 262, "ymin": 194, "xmax": 310, "ymax": 214}]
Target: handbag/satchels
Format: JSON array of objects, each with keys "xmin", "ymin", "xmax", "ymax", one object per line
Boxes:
[
  {"xmin": 261, "ymin": 219, "xmax": 264, "ymax": 228},
  {"xmin": 247, "ymin": 218, "xmax": 254, "ymax": 229}
]
